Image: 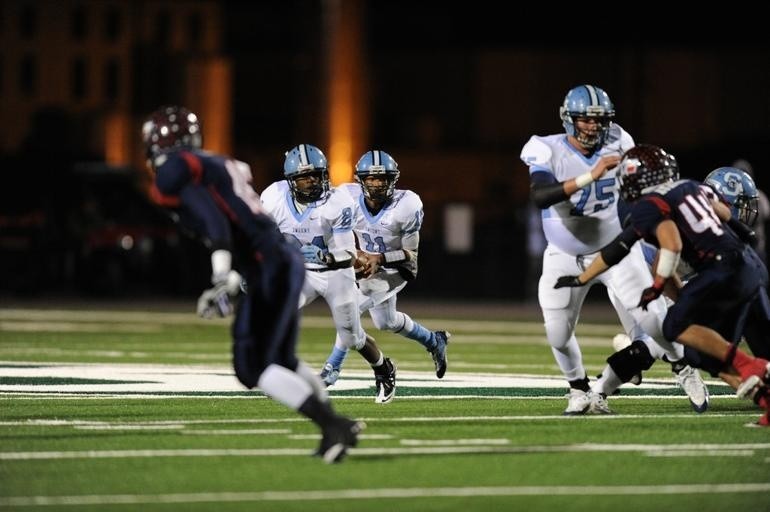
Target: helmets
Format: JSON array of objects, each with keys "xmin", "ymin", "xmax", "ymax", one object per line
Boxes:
[
  {"xmin": 559, "ymin": 85, "xmax": 616, "ymax": 151},
  {"xmin": 703, "ymin": 167, "xmax": 759, "ymax": 225},
  {"xmin": 617, "ymin": 145, "xmax": 678, "ymax": 202},
  {"xmin": 142, "ymin": 105, "xmax": 202, "ymax": 170},
  {"xmin": 353, "ymin": 150, "xmax": 400, "ymax": 202},
  {"xmin": 284, "ymin": 144, "xmax": 331, "ymax": 204}
]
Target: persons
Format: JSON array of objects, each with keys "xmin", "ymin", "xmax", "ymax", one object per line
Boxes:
[
  {"xmin": 191, "ymin": 141, "xmax": 399, "ymax": 406},
  {"xmin": 519, "ymin": 82, "xmax": 712, "ymax": 419},
  {"xmin": 315, "ymin": 146, "xmax": 452, "ymax": 390},
  {"xmin": 60, "ymin": 193, "xmax": 123, "ymax": 297},
  {"xmin": 134, "ymin": 104, "xmax": 368, "ymax": 463},
  {"xmin": 550, "ymin": 141, "xmax": 770, "ymax": 427}
]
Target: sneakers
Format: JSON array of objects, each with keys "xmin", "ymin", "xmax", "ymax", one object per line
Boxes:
[
  {"xmin": 676, "ymin": 364, "xmax": 709, "ymax": 413},
  {"xmin": 737, "ymin": 357, "xmax": 770, "ymax": 400},
  {"xmin": 428, "ymin": 330, "xmax": 451, "ymax": 379},
  {"xmin": 564, "ymin": 388, "xmax": 611, "ymax": 415},
  {"xmin": 316, "ymin": 417, "xmax": 367, "ymax": 463},
  {"xmin": 375, "ymin": 357, "xmax": 396, "ymax": 404},
  {"xmin": 613, "ymin": 333, "xmax": 642, "ymax": 385},
  {"xmin": 319, "ymin": 361, "xmax": 341, "ymax": 386}
]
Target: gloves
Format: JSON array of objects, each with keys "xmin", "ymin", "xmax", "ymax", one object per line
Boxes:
[
  {"xmin": 554, "ymin": 276, "xmax": 584, "ymax": 288},
  {"xmin": 637, "ymin": 281, "xmax": 665, "ymax": 311}
]
